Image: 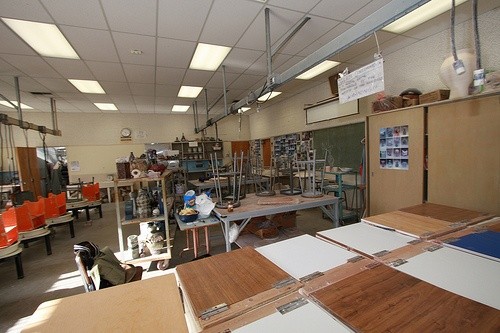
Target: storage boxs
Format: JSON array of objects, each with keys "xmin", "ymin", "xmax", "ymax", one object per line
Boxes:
[{"xmin": 369, "ymin": 90, "xmax": 450, "ymax": 113}]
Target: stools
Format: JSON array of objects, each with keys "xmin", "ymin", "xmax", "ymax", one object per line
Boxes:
[
  {"xmin": 209, "ymin": 149, "xmax": 328, "ymax": 212},
  {"xmin": 0, "ymin": 193, "xmax": 103, "ymax": 280}
]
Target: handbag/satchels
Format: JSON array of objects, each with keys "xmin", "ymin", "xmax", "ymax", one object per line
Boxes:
[
  {"xmin": 195, "ymin": 192, "xmax": 217, "ymax": 219},
  {"xmin": 91, "ymin": 245, "xmax": 137, "ymax": 286}
]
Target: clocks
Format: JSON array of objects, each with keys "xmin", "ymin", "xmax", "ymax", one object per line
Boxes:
[{"xmin": 121, "ymin": 128, "xmax": 131, "ymax": 137}]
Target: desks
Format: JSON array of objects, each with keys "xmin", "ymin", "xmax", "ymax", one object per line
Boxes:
[
  {"xmin": 208, "ymin": 189, "xmax": 337, "ymax": 253},
  {"xmin": 188, "ymin": 175, "xmax": 270, "ymax": 196},
  {"xmin": 66, "ymin": 177, "xmax": 134, "ymax": 204},
  {"xmin": 215, "ymin": 172, "xmax": 246, "ymax": 177},
  {"xmin": 173, "ymin": 210, "xmax": 220, "ymax": 258}
]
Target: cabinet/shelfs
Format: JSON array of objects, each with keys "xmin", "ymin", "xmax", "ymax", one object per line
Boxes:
[
  {"xmin": 320, "ymin": 168, "xmax": 366, "ymax": 224},
  {"xmin": 114, "ymin": 168, "xmax": 173, "ymax": 262},
  {"xmin": 171, "ymin": 140, "xmax": 224, "ymax": 161}
]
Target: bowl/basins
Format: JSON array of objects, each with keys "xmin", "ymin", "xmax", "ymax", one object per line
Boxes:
[{"xmin": 177, "ymin": 210, "xmax": 199, "ymax": 223}]
